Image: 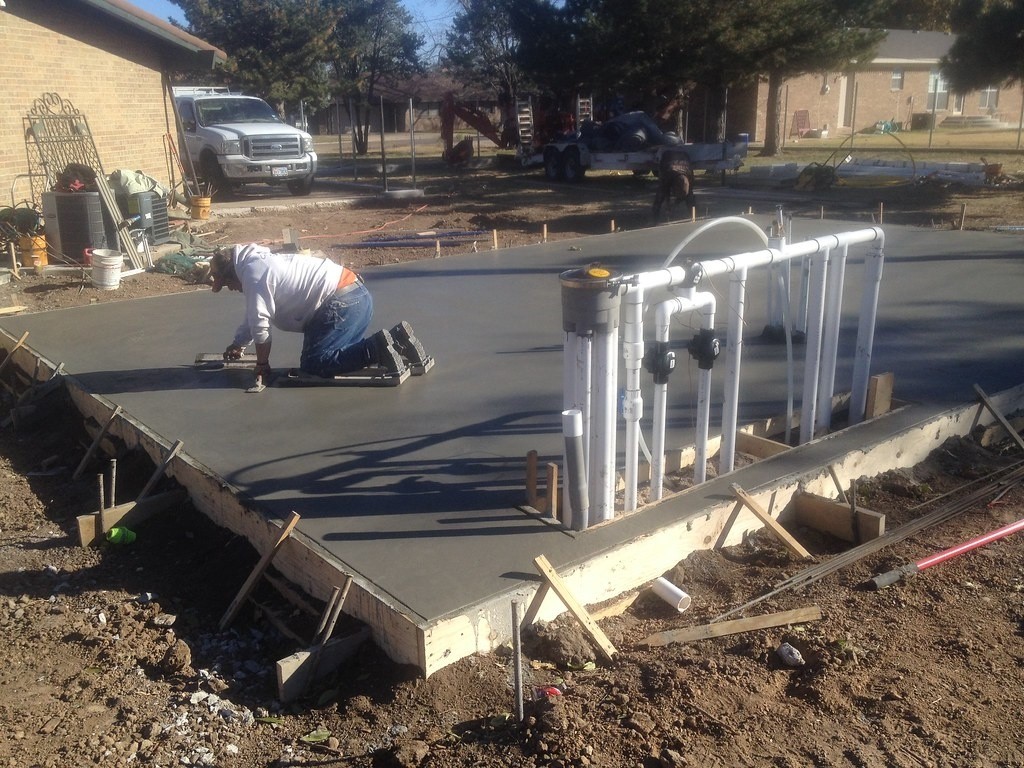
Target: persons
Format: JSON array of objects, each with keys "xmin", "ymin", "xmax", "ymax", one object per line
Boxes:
[
  {"xmin": 206, "ymin": 243, "xmax": 428, "ymax": 378},
  {"xmin": 651, "ymin": 150, "xmax": 697, "ymax": 222}
]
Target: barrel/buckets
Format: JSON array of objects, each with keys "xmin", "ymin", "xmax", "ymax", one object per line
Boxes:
[
  {"xmin": 191, "ymin": 196, "xmax": 211, "ymax": 220},
  {"xmin": 91, "ymin": 249, "xmax": 123, "ymax": 290},
  {"xmin": 21, "ymin": 235, "xmax": 47, "ymax": 267}
]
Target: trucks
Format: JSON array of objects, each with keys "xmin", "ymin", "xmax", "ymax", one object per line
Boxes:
[{"xmin": 538, "ymin": 128, "xmax": 748, "ymax": 183}]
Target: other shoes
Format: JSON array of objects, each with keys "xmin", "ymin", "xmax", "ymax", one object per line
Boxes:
[
  {"xmin": 363, "ymin": 329, "xmax": 406, "ymax": 377},
  {"xmin": 387, "ymin": 321, "xmax": 428, "ymax": 366}
]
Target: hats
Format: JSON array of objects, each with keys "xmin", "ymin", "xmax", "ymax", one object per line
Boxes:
[{"xmin": 210, "ymin": 248, "xmax": 234, "ymax": 292}]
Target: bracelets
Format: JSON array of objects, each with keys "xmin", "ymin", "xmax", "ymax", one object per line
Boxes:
[{"xmin": 257, "ymin": 360, "xmax": 268, "ymax": 366}]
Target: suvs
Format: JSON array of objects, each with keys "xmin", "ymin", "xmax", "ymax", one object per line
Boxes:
[{"xmin": 168, "ymin": 93, "xmax": 319, "ymax": 201}]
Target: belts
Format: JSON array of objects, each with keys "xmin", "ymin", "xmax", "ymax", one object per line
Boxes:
[{"xmin": 331, "ymin": 280, "xmax": 364, "ymax": 300}]
